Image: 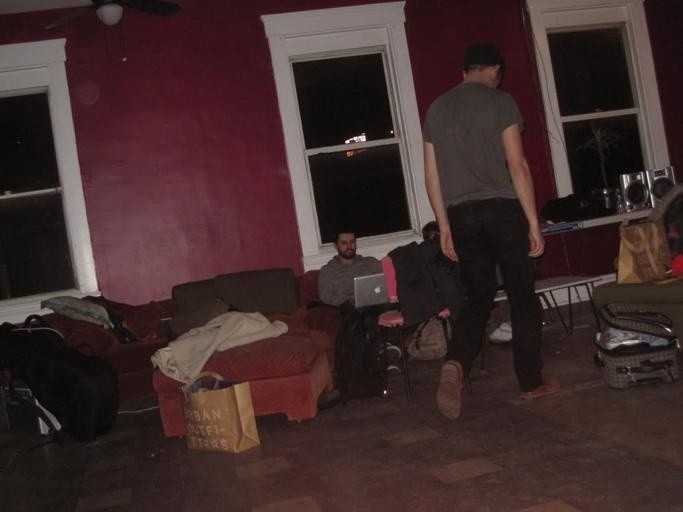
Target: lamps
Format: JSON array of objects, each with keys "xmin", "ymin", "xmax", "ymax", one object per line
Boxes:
[{"xmin": 96, "ymin": 4, "xmax": 124, "ymax": 28}]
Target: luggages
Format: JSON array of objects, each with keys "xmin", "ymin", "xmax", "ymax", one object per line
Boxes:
[{"xmin": 592, "ymin": 302, "xmax": 682, "ymax": 391}]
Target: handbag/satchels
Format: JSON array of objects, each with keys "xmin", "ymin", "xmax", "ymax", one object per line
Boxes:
[{"xmin": 615, "ymin": 218, "xmax": 672, "ymax": 284}]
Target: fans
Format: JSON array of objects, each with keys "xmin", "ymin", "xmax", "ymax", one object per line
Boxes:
[{"xmin": 43, "ymin": 0, "xmax": 182, "ymax": 32}]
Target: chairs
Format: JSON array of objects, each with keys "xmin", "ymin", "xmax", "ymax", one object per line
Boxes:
[{"xmin": 377, "ymin": 256, "xmax": 472, "ymax": 406}]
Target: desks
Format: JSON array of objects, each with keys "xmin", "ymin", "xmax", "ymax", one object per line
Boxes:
[{"xmin": 479, "ymin": 274, "xmax": 604, "ymax": 370}]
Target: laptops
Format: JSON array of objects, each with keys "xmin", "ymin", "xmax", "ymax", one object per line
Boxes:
[{"xmin": 353, "ymin": 273, "xmax": 388, "ymax": 308}]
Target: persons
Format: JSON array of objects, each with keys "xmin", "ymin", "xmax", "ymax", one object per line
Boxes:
[
  {"xmin": 422, "ymin": 219, "xmax": 463, "ymax": 321},
  {"xmin": 420, "ymin": 39, "xmax": 562, "ymax": 420},
  {"xmin": 317, "ymin": 224, "xmax": 397, "ymax": 407}
]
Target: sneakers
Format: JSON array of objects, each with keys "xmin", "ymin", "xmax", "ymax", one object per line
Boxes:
[
  {"xmin": 377, "ymin": 343, "xmax": 402, "ymax": 377},
  {"xmin": 436, "ymin": 363, "xmax": 463, "ymax": 420},
  {"xmin": 520, "ymin": 376, "xmax": 560, "ymax": 401},
  {"xmin": 489, "ymin": 322, "xmax": 514, "ymax": 343}
]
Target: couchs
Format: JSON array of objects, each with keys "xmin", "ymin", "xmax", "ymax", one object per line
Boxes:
[{"xmin": 61, "ymin": 266, "xmax": 346, "ymax": 415}]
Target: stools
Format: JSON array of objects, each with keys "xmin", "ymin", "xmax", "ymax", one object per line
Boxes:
[{"xmin": 152, "ymin": 329, "xmax": 337, "ymax": 440}]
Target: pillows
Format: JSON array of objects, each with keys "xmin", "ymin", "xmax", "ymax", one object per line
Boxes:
[
  {"xmin": 40, "ymin": 295, "xmax": 114, "ymax": 329},
  {"xmin": 173, "ymin": 268, "xmax": 300, "ymax": 326}
]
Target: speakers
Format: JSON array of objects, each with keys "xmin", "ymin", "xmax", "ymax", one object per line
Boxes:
[
  {"xmin": 645, "ymin": 165, "xmax": 675, "ymax": 209},
  {"xmin": 619, "ymin": 171, "xmax": 652, "ymax": 212}
]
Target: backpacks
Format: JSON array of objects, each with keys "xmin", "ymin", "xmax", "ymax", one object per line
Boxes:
[{"xmin": 407, "ymin": 315, "xmax": 453, "ymax": 361}]
